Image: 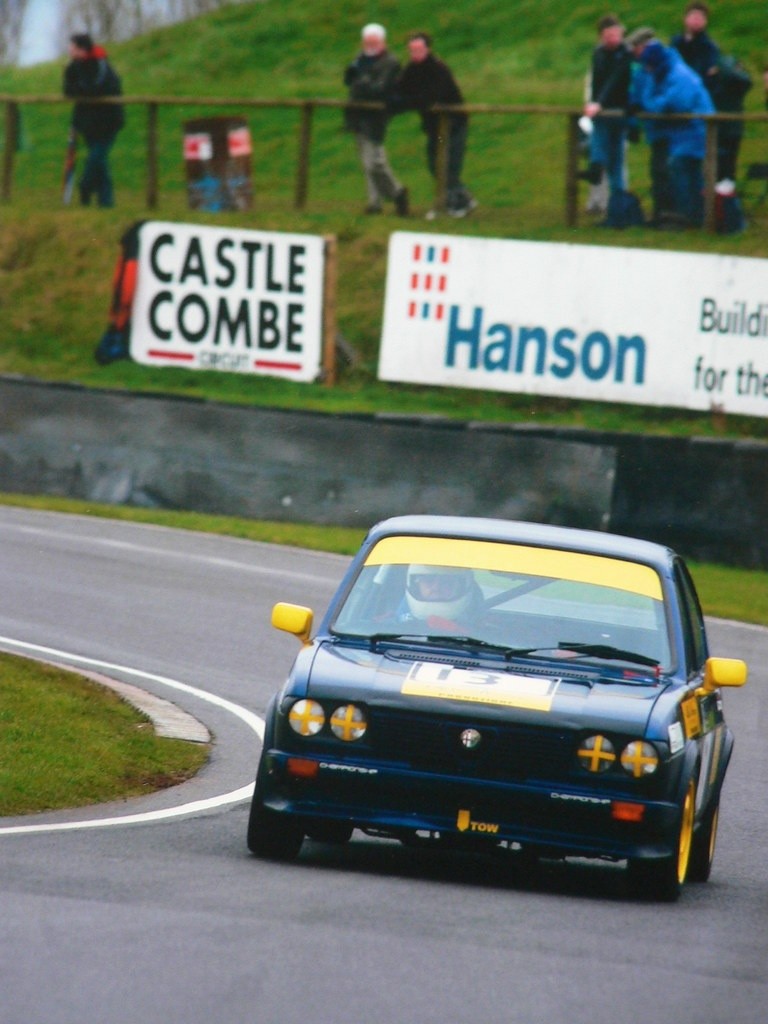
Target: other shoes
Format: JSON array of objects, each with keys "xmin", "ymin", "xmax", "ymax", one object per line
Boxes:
[
  {"xmin": 451, "ymin": 202, "xmax": 479, "ymax": 219},
  {"xmin": 364, "ymin": 202, "xmax": 382, "ymax": 216},
  {"xmin": 396, "ymin": 190, "xmax": 411, "ymax": 217},
  {"xmin": 425, "ymin": 205, "xmax": 439, "ymax": 221}
]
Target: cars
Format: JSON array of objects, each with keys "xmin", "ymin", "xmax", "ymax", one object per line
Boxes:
[{"xmin": 246, "ymin": 514, "xmax": 748, "ymax": 901}]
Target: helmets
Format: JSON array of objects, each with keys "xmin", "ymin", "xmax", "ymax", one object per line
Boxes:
[{"xmin": 404, "ymin": 563, "xmax": 474, "ymax": 623}]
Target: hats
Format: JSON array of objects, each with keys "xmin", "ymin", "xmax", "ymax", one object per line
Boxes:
[{"xmin": 361, "ymin": 23, "xmax": 387, "ymax": 58}]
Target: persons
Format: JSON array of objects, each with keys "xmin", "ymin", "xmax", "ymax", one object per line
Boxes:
[
  {"xmin": 382, "ymin": 32, "xmax": 480, "ymax": 217},
  {"xmin": 339, "ymin": 21, "xmax": 410, "ymax": 216},
  {"xmin": 62, "ymin": 34, "xmax": 126, "ymax": 207},
  {"xmin": 583, "ymin": 4, "xmax": 751, "ymax": 234},
  {"xmin": 381, "ymin": 562, "xmax": 493, "ymax": 644}
]
